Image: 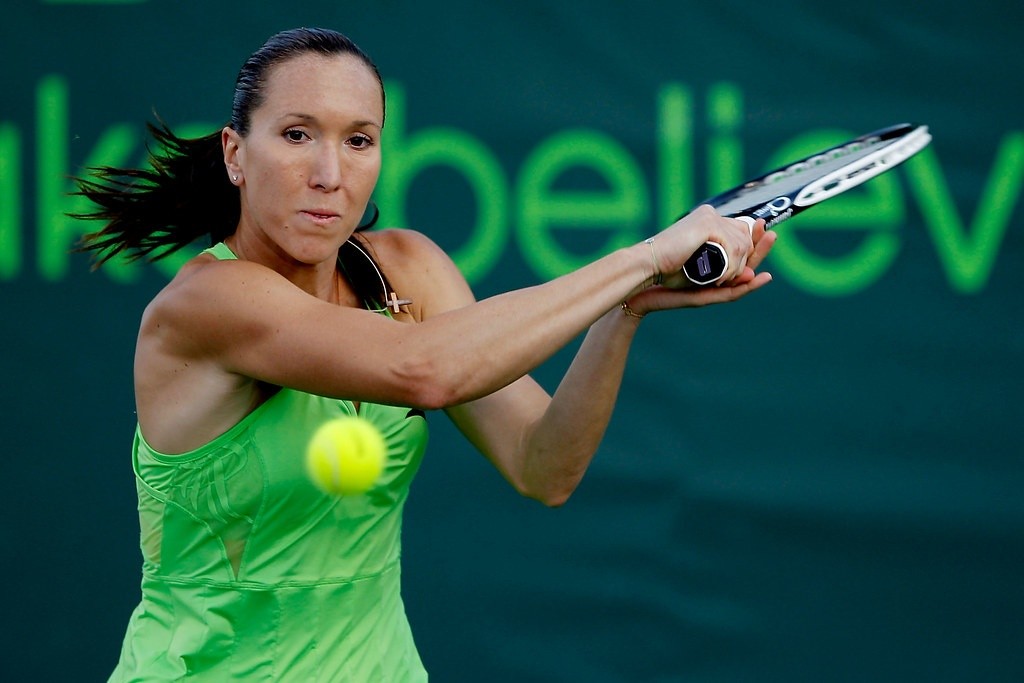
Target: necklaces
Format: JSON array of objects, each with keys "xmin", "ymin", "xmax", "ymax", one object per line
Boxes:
[{"xmin": 224, "ymin": 236, "xmax": 413, "ymax": 314}]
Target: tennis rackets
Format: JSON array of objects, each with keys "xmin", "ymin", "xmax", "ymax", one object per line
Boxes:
[{"xmin": 671, "ymin": 119, "xmax": 935, "ymax": 287}]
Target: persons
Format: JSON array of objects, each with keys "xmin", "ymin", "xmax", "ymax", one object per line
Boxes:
[{"xmin": 67, "ymin": 27, "xmax": 778, "ymax": 683}]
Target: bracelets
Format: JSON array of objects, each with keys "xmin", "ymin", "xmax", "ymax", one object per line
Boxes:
[
  {"xmin": 621, "ymin": 302, "xmax": 645, "ymax": 318},
  {"xmin": 645, "ymin": 237, "xmax": 660, "ymax": 271}
]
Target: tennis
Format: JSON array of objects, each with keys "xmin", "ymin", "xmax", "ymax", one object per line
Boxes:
[{"xmin": 302, "ymin": 415, "xmax": 388, "ymax": 501}]
